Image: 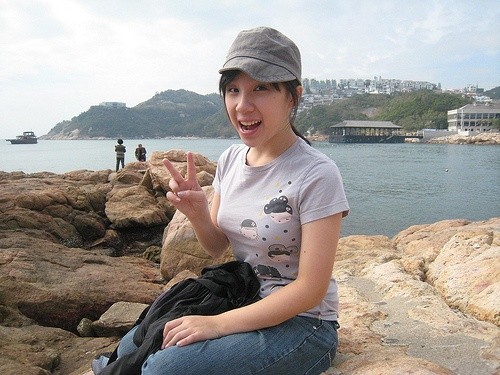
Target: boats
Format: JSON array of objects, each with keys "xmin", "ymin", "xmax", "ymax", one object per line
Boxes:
[{"xmin": 10, "ymin": 131, "xmax": 37, "ymax": 144}]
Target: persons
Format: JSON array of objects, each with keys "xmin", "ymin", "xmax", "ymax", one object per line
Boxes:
[
  {"xmin": 135, "ymin": 144, "xmax": 146, "ymax": 162},
  {"xmin": 91, "ymin": 26, "xmax": 350, "ymax": 375},
  {"xmin": 115, "ymin": 139, "xmax": 126, "ymax": 172}
]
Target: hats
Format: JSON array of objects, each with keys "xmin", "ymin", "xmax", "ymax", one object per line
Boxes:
[{"xmin": 218, "ymin": 27, "xmax": 301, "ymax": 84}]
